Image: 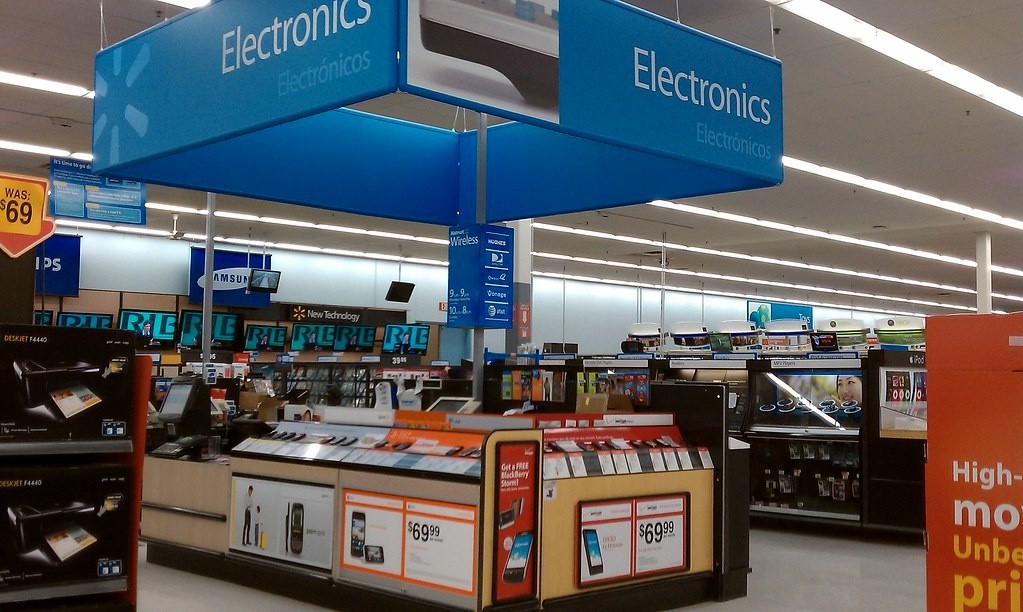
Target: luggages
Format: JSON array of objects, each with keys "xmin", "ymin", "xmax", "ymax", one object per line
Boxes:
[{"xmin": 260, "ymin": 523, "xmax": 266, "ymax": 550}]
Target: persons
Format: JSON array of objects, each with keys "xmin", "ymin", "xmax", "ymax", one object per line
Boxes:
[
  {"xmin": 253, "ymin": 506, "xmax": 263, "ymax": 548},
  {"xmin": 603, "ymin": 378, "xmax": 609, "ymax": 393},
  {"xmin": 596, "ymin": 377, "xmax": 606, "ymax": 392},
  {"xmin": 393, "ymin": 333, "xmax": 411, "ymax": 356},
  {"xmin": 255, "ymin": 334, "xmax": 270, "ymax": 351},
  {"xmin": 193, "ymin": 329, "xmax": 214, "ymax": 348},
  {"xmin": 241, "ymin": 485, "xmax": 254, "ymax": 547},
  {"xmin": 295, "ymin": 366, "xmax": 305, "ymax": 378},
  {"xmin": 637, "ymin": 389, "xmax": 646, "ymax": 402},
  {"xmin": 343, "ymin": 334, "xmax": 360, "ymax": 352},
  {"xmin": 136, "ymin": 320, "xmax": 154, "ymax": 349},
  {"xmin": 616, "ymin": 377, "xmax": 624, "ymax": 395},
  {"xmin": 302, "ymin": 331, "xmax": 319, "ymax": 351},
  {"xmin": 304, "ymin": 410, "xmax": 312, "ymax": 421},
  {"xmin": 543, "ymin": 376, "xmax": 552, "ymax": 402},
  {"xmin": 836, "ymin": 375, "xmax": 862, "ymax": 409}
]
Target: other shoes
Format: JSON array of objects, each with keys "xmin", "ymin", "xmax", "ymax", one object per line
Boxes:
[
  {"xmin": 254, "ymin": 544, "xmax": 258, "ymax": 547},
  {"xmin": 246, "ymin": 541, "xmax": 251, "ymax": 545},
  {"xmin": 242, "ymin": 542, "xmax": 247, "ymax": 546}
]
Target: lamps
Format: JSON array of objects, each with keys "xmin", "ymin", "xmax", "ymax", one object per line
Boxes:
[{"xmin": 0, "ymin": 0, "xmax": 1023, "ymax": 324}]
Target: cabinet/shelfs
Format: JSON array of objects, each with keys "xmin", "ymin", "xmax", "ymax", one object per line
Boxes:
[{"xmin": 0, "ymin": 312, "xmax": 1023, "ymax": 610}]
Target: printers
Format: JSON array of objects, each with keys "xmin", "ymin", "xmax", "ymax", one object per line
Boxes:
[
  {"xmin": 12, "ymin": 357, "xmax": 104, "ymax": 422},
  {"xmin": 7, "ymin": 498, "xmax": 102, "ymax": 568}
]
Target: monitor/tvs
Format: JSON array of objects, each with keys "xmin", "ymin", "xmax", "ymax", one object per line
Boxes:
[
  {"xmin": 57, "ymin": 312, "xmax": 114, "ymax": 328},
  {"xmin": 178, "ymin": 309, "xmax": 239, "ymax": 349},
  {"xmin": 290, "ymin": 323, "xmax": 378, "ymax": 353},
  {"xmin": 425, "ymin": 397, "xmax": 475, "ymax": 413},
  {"xmin": 381, "ymin": 324, "xmax": 430, "ymax": 356},
  {"xmin": 242, "ymin": 324, "xmax": 288, "ymax": 353},
  {"xmin": 277, "ymin": 390, "xmax": 310, "ymax": 401},
  {"xmin": 206, "ymin": 378, "xmax": 239, "ymax": 412},
  {"xmin": 247, "ymin": 268, "xmax": 281, "ymax": 293},
  {"xmin": 158, "ymin": 376, "xmax": 199, "ymax": 421},
  {"xmin": 34, "ymin": 310, "xmax": 54, "ymax": 326},
  {"xmin": 117, "ymin": 308, "xmax": 179, "ymax": 350}
]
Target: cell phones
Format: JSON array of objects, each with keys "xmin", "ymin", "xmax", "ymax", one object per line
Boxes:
[
  {"xmin": 499, "ymin": 508, "xmax": 514, "ymax": 530},
  {"xmin": 286, "ymin": 502, "xmax": 304, "ymax": 553},
  {"xmin": 582, "ymin": 529, "xmax": 604, "ymax": 576},
  {"xmin": 502, "ymin": 531, "xmax": 534, "ymax": 583},
  {"xmin": 351, "ymin": 511, "xmax": 385, "ymax": 564}
]
